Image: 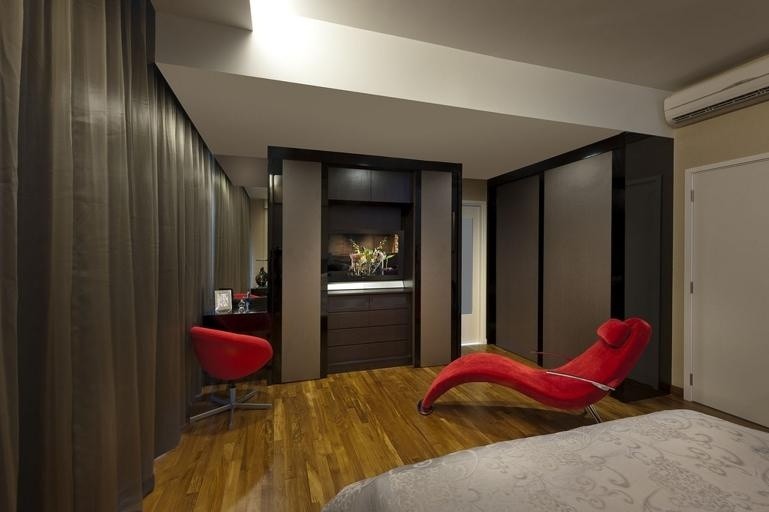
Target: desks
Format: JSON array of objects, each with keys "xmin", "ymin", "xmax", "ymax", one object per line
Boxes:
[{"xmin": 201, "ymin": 313, "xmax": 274, "ymax": 344}]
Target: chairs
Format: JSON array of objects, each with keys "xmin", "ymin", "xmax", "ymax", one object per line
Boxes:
[
  {"xmin": 417, "ymin": 319, "xmax": 653, "ymax": 423},
  {"xmin": 185, "ymin": 326, "xmax": 272, "ymax": 428}
]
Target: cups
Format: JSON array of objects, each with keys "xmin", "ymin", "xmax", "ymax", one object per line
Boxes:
[{"xmin": 238, "ymin": 299, "xmax": 246, "ymax": 313}]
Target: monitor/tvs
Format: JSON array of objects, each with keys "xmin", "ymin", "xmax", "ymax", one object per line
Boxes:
[{"xmin": 327, "ymin": 230, "xmax": 405, "ymax": 291}]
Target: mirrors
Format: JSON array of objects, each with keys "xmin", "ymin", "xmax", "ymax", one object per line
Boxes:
[{"xmin": 212, "ymin": 154, "xmax": 268, "ymax": 316}]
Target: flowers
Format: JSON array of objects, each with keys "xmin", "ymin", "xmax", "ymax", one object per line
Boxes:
[{"xmin": 347, "ymin": 234, "xmax": 389, "ymax": 278}]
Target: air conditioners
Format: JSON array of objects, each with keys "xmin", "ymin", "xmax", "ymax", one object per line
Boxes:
[{"xmin": 663, "ymin": 53, "xmax": 769, "ymax": 129}]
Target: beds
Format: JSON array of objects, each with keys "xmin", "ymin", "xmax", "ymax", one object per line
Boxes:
[{"xmin": 316, "ymin": 406, "xmax": 769, "ymax": 512}]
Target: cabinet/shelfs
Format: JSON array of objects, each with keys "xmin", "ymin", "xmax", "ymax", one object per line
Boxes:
[{"xmin": 325, "ymin": 293, "xmax": 412, "ymax": 374}]
